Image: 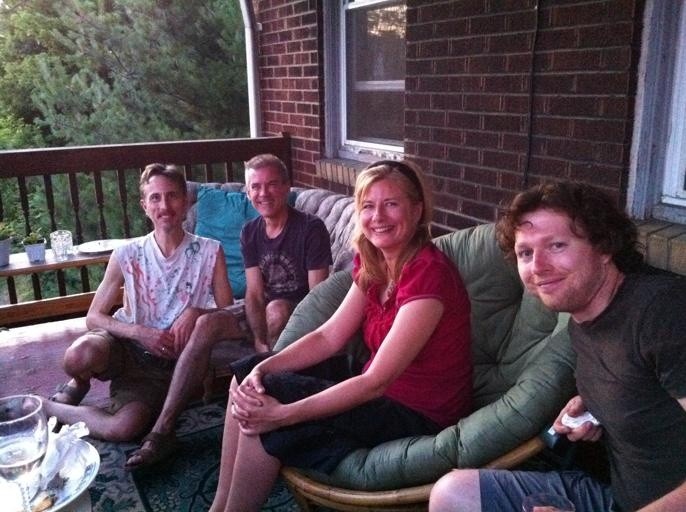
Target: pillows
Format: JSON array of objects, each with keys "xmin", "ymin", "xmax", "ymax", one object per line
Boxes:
[{"xmin": 194, "ymin": 186, "xmax": 298, "ymax": 299}]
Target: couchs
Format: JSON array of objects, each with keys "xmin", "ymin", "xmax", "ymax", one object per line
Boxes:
[{"xmin": 182, "ymin": 179, "xmax": 363, "ymax": 406}]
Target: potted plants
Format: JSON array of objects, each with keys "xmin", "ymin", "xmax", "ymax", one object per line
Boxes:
[
  {"xmin": 0, "ymin": 219, "xmax": 10, "ymax": 266},
  {"xmin": 23, "ymin": 232, "xmax": 47, "ymax": 263}
]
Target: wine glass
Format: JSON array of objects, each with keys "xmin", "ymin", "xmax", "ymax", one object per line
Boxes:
[{"xmin": 0, "ymin": 394, "xmax": 50, "ymax": 512}]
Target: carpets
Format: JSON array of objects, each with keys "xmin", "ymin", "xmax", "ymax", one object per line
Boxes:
[{"xmin": 90, "ymin": 395, "xmax": 305, "ymax": 512}]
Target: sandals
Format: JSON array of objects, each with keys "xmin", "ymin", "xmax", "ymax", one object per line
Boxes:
[
  {"xmin": 45, "ymin": 382, "xmax": 91, "ymax": 406},
  {"xmin": 0, "ymin": 394, "xmax": 31, "ymax": 436},
  {"xmin": 124, "ymin": 433, "xmax": 167, "ymax": 471}
]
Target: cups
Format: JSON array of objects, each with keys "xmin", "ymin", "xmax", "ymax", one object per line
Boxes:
[{"xmin": 50, "ymin": 230, "xmax": 74, "ymax": 262}]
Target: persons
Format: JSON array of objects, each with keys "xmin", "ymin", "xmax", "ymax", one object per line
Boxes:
[
  {"xmin": 123, "ymin": 154, "xmax": 333, "ymax": 473},
  {"xmin": 207, "ymin": 158, "xmax": 473, "ymax": 512},
  {"xmin": 0, "ymin": 163, "xmax": 235, "ymax": 443},
  {"xmin": 427, "ymin": 183, "xmax": 686, "ymax": 512}
]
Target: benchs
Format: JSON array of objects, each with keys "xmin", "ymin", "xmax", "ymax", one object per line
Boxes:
[{"xmin": 0, "ymin": 251, "xmax": 111, "ymax": 277}]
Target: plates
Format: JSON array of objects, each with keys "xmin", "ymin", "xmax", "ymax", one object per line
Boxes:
[
  {"xmin": 0, "ymin": 430, "xmax": 102, "ymax": 512},
  {"xmin": 77, "ymin": 238, "xmax": 123, "ymax": 255}
]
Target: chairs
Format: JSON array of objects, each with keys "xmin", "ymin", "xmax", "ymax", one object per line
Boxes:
[{"xmin": 264, "ymin": 222, "xmax": 578, "ymax": 512}]
